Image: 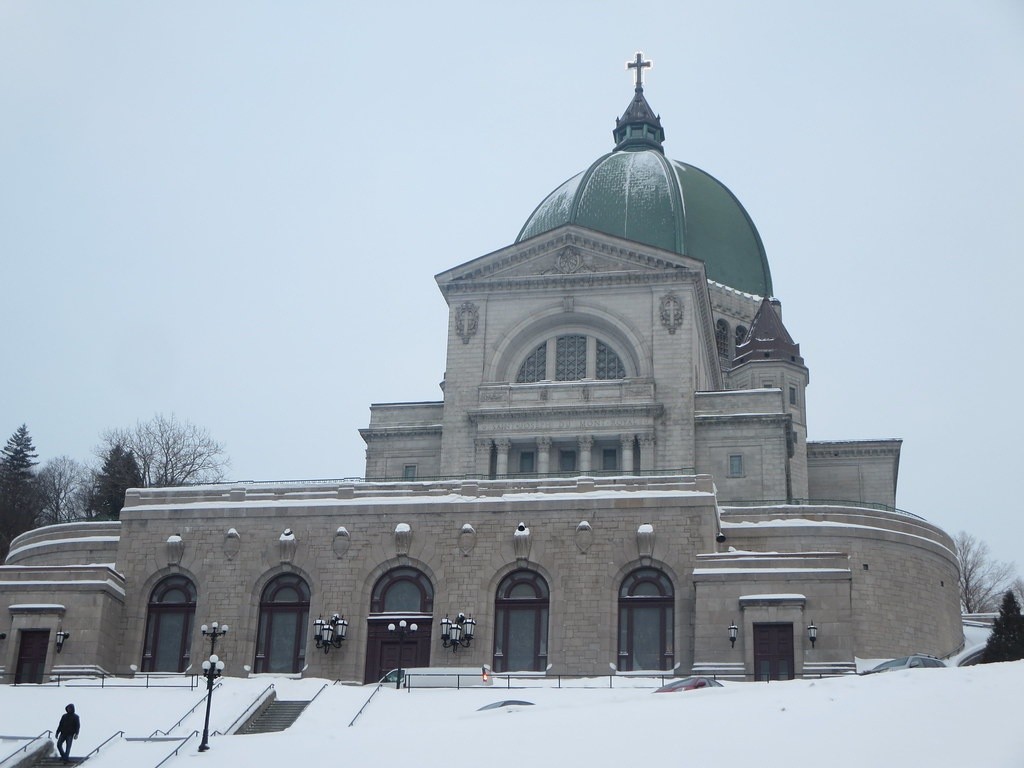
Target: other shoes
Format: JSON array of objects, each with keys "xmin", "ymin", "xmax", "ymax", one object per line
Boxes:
[{"xmin": 63, "ymin": 758, "xmax": 67, "ymax": 764}]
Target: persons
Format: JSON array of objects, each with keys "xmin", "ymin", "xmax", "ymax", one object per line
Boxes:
[{"xmin": 54, "ymin": 703, "xmax": 80, "ymax": 761}]
